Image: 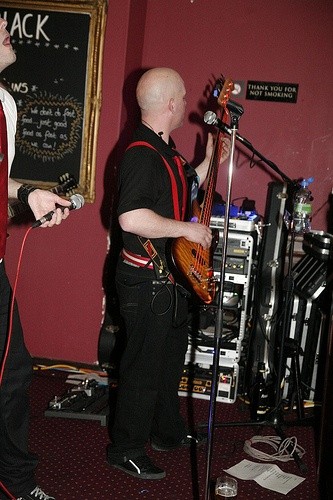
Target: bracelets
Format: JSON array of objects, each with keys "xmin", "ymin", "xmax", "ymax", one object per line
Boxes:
[{"xmin": 17, "ymin": 183, "xmax": 41, "ymax": 207}]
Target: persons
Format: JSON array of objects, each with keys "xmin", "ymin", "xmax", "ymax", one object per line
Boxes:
[
  {"xmin": 106, "ymin": 67, "xmax": 231, "ymax": 480},
  {"xmin": 0, "ymin": 15, "xmax": 71, "ymax": 500}
]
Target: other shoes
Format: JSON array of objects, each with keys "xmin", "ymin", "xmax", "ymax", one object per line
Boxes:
[
  {"xmin": 151, "ymin": 432, "xmax": 208, "ymax": 452},
  {"xmin": 106, "ymin": 452, "xmax": 166, "ymax": 479},
  {"xmin": 17, "ymin": 486, "xmax": 57, "ymax": 500}
]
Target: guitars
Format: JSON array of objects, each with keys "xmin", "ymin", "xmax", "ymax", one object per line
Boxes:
[{"xmin": 165, "ymin": 73, "xmax": 243, "ymax": 305}]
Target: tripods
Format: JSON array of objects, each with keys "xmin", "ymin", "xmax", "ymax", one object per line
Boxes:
[{"xmin": 201, "ymin": 143, "xmax": 315, "ymax": 473}]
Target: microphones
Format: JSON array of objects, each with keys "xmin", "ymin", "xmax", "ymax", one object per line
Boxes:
[
  {"xmin": 203, "ymin": 111, "xmax": 253, "ymax": 148},
  {"xmin": 32, "ymin": 194, "xmax": 85, "ymax": 228}
]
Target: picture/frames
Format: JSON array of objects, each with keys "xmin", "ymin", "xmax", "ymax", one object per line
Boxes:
[{"xmin": 0, "ymin": 0, "xmax": 109, "ymax": 204}]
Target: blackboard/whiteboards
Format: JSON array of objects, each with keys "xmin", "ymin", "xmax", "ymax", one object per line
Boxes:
[{"xmin": 0, "ymin": 0, "xmax": 108, "ymax": 207}]
